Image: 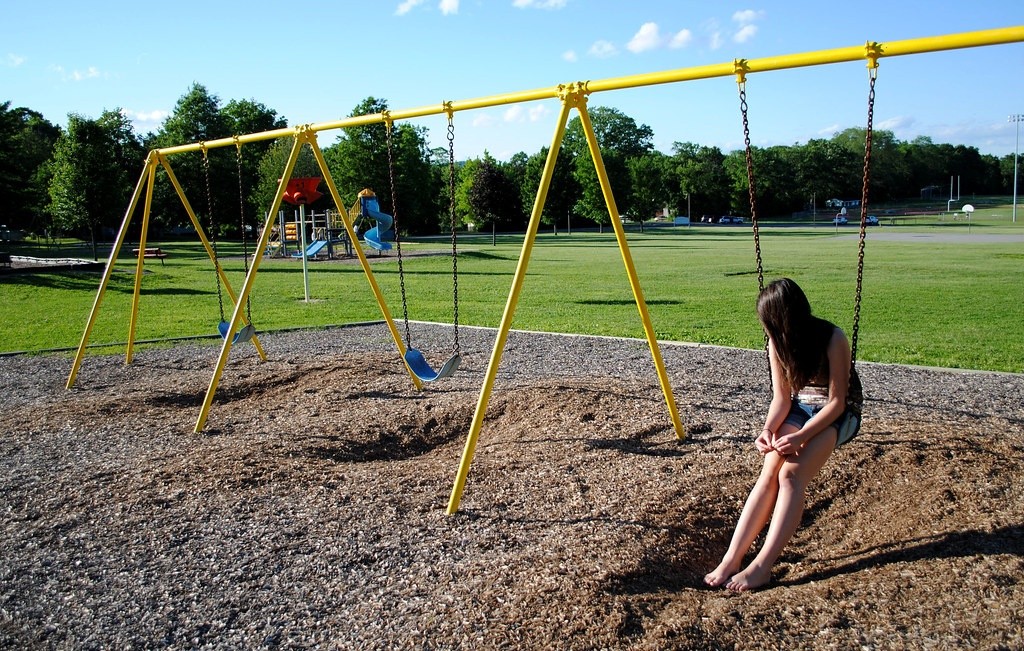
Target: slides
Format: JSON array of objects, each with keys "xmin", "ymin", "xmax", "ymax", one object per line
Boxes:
[
  {"xmin": 290, "ymin": 239, "xmax": 330, "ymax": 259},
  {"xmin": 361, "ymin": 194, "xmax": 394, "ymax": 251}
]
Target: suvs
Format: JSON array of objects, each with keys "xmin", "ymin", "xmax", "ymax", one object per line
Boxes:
[
  {"xmin": 866, "ymin": 216, "xmax": 878, "ymax": 226},
  {"xmin": 832, "ymin": 217, "xmax": 848, "ymax": 225}
]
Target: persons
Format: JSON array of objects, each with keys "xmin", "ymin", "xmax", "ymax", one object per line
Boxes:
[{"xmin": 701, "ymin": 276, "xmax": 863, "ymax": 593}]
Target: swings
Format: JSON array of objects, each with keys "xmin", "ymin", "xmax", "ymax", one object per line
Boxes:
[
  {"xmin": 383, "ymin": 117, "xmax": 463, "ymax": 382},
  {"xmin": 198, "ymin": 141, "xmax": 256, "ymax": 344},
  {"xmin": 734, "ymin": 61, "xmax": 880, "ymax": 450}
]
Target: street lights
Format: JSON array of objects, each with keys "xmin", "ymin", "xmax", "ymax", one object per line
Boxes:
[{"xmin": 1007, "ymin": 114, "xmax": 1024, "ymax": 223}]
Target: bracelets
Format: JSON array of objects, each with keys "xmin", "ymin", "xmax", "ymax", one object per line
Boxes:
[{"xmin": 760, "ymin": 428, "xmax": 773, "ymax": 436}]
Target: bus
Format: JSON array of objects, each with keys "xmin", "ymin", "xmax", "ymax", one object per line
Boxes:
[{"xmin": 719, "ymin": 216, "xmax": 744, "ymax": 223}]
